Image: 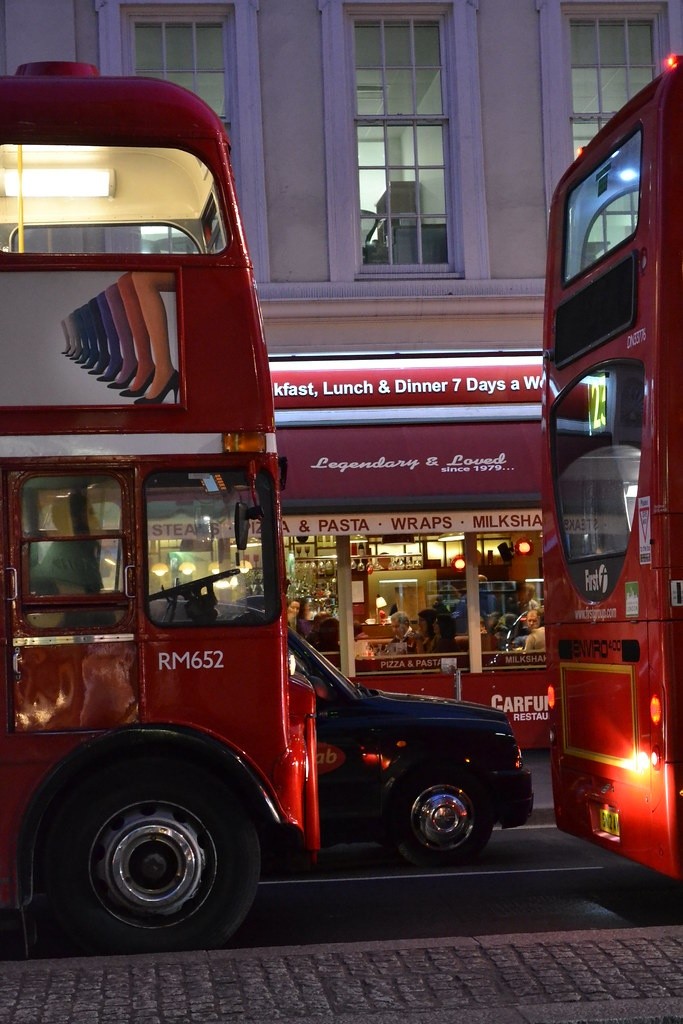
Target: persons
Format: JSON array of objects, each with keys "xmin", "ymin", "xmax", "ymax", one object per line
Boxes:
[
  {"xmin": 59, "ymin": 272, "xmax": 179, "ymax": 403},
  {"xmin": 30, "ymin": 484, "xmax": 120, "ymax": 626},
  {"xmin": 382, "ymin": 573, "xmax": 546, "ymax": 656},
  {"xmin": 285, "ymin": 598, "xmax": 340, "ymax": 666}
]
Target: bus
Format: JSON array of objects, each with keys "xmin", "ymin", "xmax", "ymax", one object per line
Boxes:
[
  {"xmin": 0, "ymin": 61, "xmax": 320, "ymax": 958},
  {"xmin": 39, "ymin": 351, "xmax": 551, "ymax": 750},
  {"xmin": 541, "ymin": 57, "xmax": 683, "ymax": 879}
]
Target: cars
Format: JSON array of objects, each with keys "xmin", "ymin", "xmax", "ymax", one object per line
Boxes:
[{"xmin": 148, "ymin": 601, "xmax": 532, "ymax": 866}]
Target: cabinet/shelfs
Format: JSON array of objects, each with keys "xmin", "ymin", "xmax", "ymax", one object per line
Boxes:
[{"xmin": 376, "ymin": 181, "xmax": 422, "ymax": 243}]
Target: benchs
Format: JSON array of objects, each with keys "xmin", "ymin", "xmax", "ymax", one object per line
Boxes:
[{"xmin": 9, "ymin": 220, "xmax": 204, "ymax": 253}]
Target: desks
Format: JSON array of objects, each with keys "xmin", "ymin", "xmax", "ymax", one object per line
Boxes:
[{"xmin": 362, "ymin": 622, "xmax": 419, "ymax": 635}]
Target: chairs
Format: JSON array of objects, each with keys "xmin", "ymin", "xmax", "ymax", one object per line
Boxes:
[{"xmin": 70, "ymin": 491, "xmax": 105, "ymax": 593}]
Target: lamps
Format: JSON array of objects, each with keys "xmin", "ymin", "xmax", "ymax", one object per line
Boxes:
[
  {"xmin": 230, "ymin": 537, "xmax": 262, "ymax": 547},
  {"xmin": 179, "ymin": 562, "xmax": 195, "ymax": 575},
  {"xmin": 151, "ymin": 563, "xmax": 168, "ymax": 577},
  {"xmin": 498, "ymin": 541, "xmax": 514, "ymax": 563},
  {"xmin": 208, "ymin": 562, "xmax": 219, "ymax": 574},
  {"xmin": 375, "ymin": 594, "xmax": 388, "ymax": 624},
  {"xmin": 437, "ymin": 531, "xmax": 465, "ymax": 542},
  {"xmin": 236, "ymin": 560, "xmax": 252, "ymax": 573},
  {"xmin": 349, "ymin": 534, "xmax": 368, "ymax": 544}
]
